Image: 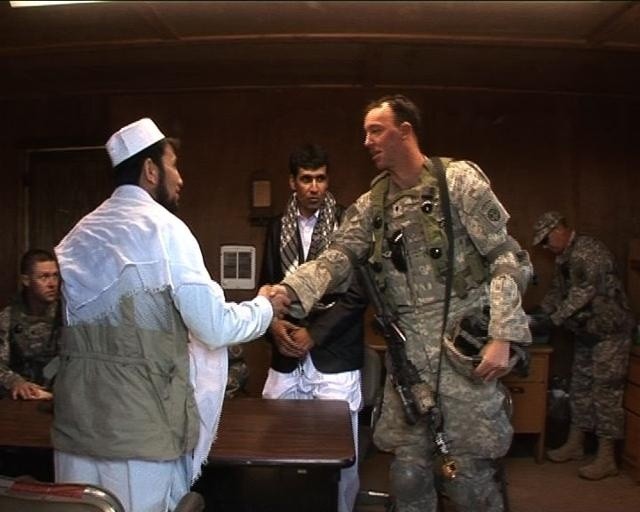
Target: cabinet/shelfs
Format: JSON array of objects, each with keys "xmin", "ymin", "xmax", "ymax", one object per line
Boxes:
[
  {"xmin": 503, "ymin": 347, "xmax": 555, "ymax": 464},
  {"xmin": 620, "ymin": 346, "xmax": 640, "ymax": 484}
]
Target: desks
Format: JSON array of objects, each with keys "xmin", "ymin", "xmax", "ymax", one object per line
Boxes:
[{"xmin": 0, "ymin": 396, "xmax": 356, "ymax": 512}]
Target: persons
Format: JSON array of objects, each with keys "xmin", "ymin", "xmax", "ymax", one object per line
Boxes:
[
  {"xmin": 261, "ymin": 146, "xmax": 369, "ymax": 512},
  {"xmin": 49, "ymin": 115, "xmax": 292, "ymax": 511},
  {"xmin": 225, "ymin": 344, "xmax": 251, "ymax": 398},
  {"xmin": 0, "ymin": 247, "xmax": 62, "ymax": 400},
  {"xmin": 531, "ymin": 210, "xmax": 636, "ymax": 481},
  {"xmin": 269, "ymin": 91, "xmax": 535, "ymax": 510}
]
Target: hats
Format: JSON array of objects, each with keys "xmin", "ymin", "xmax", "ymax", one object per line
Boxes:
[
  {"xmin": 106, "ymin": 117, "xmax": 165, "ymax": 167},
  {"xmin": 532, "ymin": 213, "xmax": 560, "ymax": 245}
]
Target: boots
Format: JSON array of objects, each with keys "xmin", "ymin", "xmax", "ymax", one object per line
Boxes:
[
  {"xmin": 548, "ymin": 429, "xmax": 585, "ymax": 461},
  {"xmin": 580, "ymin": 438, "xmax": 618, "ymax": 480}
]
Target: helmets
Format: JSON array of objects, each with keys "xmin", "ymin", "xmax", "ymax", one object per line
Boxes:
[{"xmin": 443, "ymin": 308, "xmax": 520, "ymax": 380}]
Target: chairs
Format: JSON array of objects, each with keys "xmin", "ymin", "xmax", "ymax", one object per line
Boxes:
[{"xmin": 1, "ymin": 475, "xmax": 207, "ymax": 512}]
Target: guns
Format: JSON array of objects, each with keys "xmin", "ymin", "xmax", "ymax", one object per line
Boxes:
[{"xmin": 357, "ymin": 260, "xmax": 457, "ymax": 480}]
